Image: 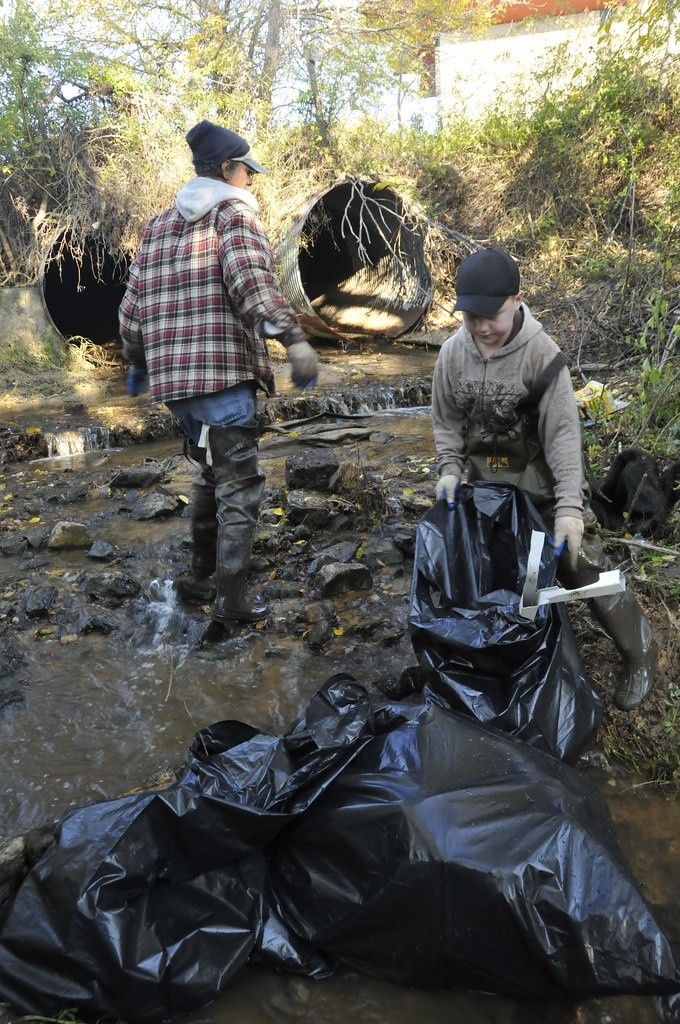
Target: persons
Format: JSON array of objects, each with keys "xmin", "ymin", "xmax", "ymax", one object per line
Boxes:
[
  {"xmin": 119, "ymin": 120, "xmax": 320, "ymax": 621},
  {"xmin": 430, "ymin": 248, "xmax": 659, "ymax": 709}
]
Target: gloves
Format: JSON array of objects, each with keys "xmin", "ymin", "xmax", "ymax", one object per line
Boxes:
[
  {"xmin": 126, "ymin": 369, "xmax": 148, "ymax": 397},
  {"xmin": 436, "ymin": 476, "xmax": 461, "ymax": 510},
  {"xmin": 287, "ymin": 341, "xmax": 321, "ymax": 390},
  {"xmin": 554, "ymin": 516, "xmax": 584, "ymax": 570}
]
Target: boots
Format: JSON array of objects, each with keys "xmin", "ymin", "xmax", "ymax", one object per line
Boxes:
[
  {"xmin": 210, "ymin": 420, "xmax": 272, "ymax": 624},
  {"xmin": 184, "ymin": 437, "xmax": 218, "ymax": 601},
  {"xmin": 536, "ymin": 479, "xmax": 663, "ymax": 712}
]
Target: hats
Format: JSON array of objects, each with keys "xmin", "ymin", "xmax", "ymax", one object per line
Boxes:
[
  {"xmin": 453, "ymin": 247, "xmax": 520, "ymax": 319},
  {"xmin": 230, "ymin": 142, "xmax": 270, "ymax": 175},
  {"xmin": 185, "ymin": 119, "xmax": 250, "ymax": 170}
]
